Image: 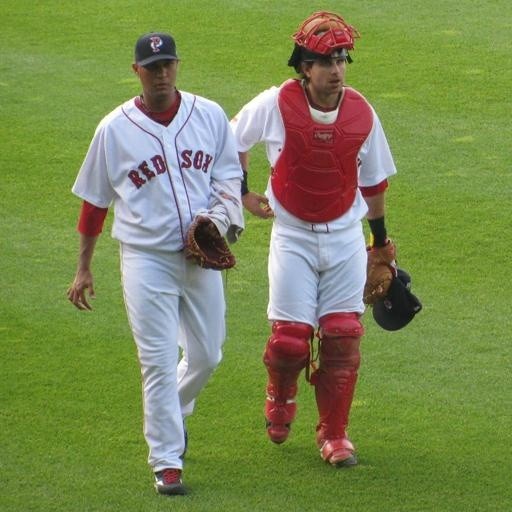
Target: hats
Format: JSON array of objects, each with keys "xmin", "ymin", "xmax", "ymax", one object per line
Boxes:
[
  {"xmin": 134, "ymin": 32, "xmax": 183, "ymax": 68},
  {"xmin": 372, "ymin": 268, "xmax": 423, "ymax": 331}
]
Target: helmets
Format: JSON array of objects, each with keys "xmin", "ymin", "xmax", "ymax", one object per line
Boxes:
[{"xmin": 288, "ymin": 10, "xmax": 361, "ymax": 74}]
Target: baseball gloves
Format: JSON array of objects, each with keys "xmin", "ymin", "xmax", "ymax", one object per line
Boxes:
[
  {"xmin": 184, "ymin": 216, "xmax": 235, "ymax": 270},
  {"xmin": 363, "ymin": 239, "xmax": 397, "ymax": 304}
]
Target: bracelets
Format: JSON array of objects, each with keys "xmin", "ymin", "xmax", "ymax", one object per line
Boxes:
[
  {"xmin": 367, "ymin": 216, "xmax": 388, "ymax": 249},
  {"xmin": 241, "ymin": 171, "xmax": 250, "ymax": 196}
]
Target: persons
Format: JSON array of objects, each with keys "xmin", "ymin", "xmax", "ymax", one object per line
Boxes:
[
  {"xmin": 230, "ymin": 11, "xmax": 398, "ymax": 468},
  {"xmin": 67, "ymin": 31, "xmax": 245, "ymax": 497}
]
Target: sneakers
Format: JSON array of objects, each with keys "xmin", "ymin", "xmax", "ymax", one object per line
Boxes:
[
  {"xmin": 153, "ymin": 469, "xmax": 186, "ymax": 495},
  {"xmin": 267, "ymin": 423, "xmax": 290, "ymax": 444},
  {"xmin": 330, "ymin": 453, "xmax": 357, "ymax": 468}
]
